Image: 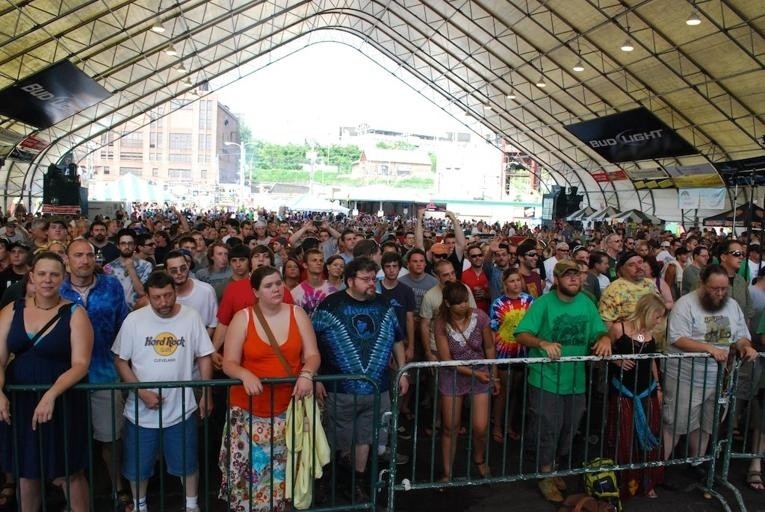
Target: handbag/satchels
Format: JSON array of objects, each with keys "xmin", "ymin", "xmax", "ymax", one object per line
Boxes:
[{"xmin": 558, "ymin": 494, "xmax": 618, "ymax": 512}]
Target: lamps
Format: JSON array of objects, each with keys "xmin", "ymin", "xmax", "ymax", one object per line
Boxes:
[
  {"xmin": 151, "ymin": 1, "xmax": 195, "ymax": 86},
  {"xmin": 464, "ymin": 0, "xmax": 703, "ymax": 117}
]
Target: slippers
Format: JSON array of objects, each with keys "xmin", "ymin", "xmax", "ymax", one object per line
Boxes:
[{"xmin": 400, "ymin": 409, "xmax": 416, "ymax": 421}]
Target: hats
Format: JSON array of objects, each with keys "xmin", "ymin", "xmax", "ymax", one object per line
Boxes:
[
  {"xmin": 431, "ymin": 243, "xmax": 450, "ymax": 254},
  {"xmin": 254, "ymin": 221, "xmax": 266, "ymax": 229},
  {"xmin": 674, "ymin": 247, "xmax": 692, "ymax": 255},
  {"xmin": 553, "ymin": 258, "xmax": 579, "ymax": 277}
]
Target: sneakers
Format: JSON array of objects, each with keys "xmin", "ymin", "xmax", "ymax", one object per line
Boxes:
[
  {"xmin": 398, "ymin": 425, "xmax": 411, "ymax": 440},
  {"xmin": 538, "ymin": 477, "xmax": 567, "ymax": 502},
  {"xmin": 378, "ymin": 446, "xmax": 409, "ymax": 464},
  {"xmin": 315, "ymin": 472, "xmax": 330, "ymax": 505},
  {"xmin": 346, "ymin": 478, "xmax": 369, "ymax": 503}
]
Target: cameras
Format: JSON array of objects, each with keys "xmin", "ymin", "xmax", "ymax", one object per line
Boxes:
[
  {"xmin": 313, "ymin": 220, "xmax": 322, "ymax": 227},
  {"xmin": 166, "ymin": 208, "xmax": 172, "ymax": 218}
]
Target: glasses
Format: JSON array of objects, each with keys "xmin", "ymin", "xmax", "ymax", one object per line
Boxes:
[
  {"xmin": 725, "ymin": 249, "xmax": 744, "ymax": 257},
  {"xmin": 471, "ymin": 253, "xmax": 483, "ymax": 258},
  {"xmin": 614, "ymin": 240, "xmax": 623, "ymax": 242},
  {"xmin": 561, "ymin": 250, "xmax": 569, "ymax": 252},
  {"xmin": 171, "ymin": 268, "xmax": 186, "ymax": 273},
  {"xmin": 629, "ymin": 242, "xmax": 635, "ymax": 245},
  {"xmin": 356, "ymin": 276, "xmax": 377, "ymax": 282},
  {"xmin": 434, "ymin": 253, "xmax": 447, "ymax": 259},
  {"xmin": 523, "ymin": 252, "xmax": 538, "ymax": 257}
]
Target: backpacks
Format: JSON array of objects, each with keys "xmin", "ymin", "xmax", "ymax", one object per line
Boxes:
[{"xmin": 583, "ymin": 459, "xmax": 623, "ymax": 511}]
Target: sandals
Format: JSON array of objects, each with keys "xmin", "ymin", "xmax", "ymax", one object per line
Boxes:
[
  {"xmin": 746, "ymin": 471, "xmax": 765, "ymax": 492},
  {"xmin": 118, "ymin": 489, "xmax": 134, "ymax": 511},
  {"xmin": 1, "ymin": 484, "xmax": 16, "ymax": 508}
]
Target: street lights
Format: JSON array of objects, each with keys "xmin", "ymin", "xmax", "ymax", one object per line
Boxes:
[{"xmin": 222, "ymin": 139, "xmax": 261, "ymax": 207}]
